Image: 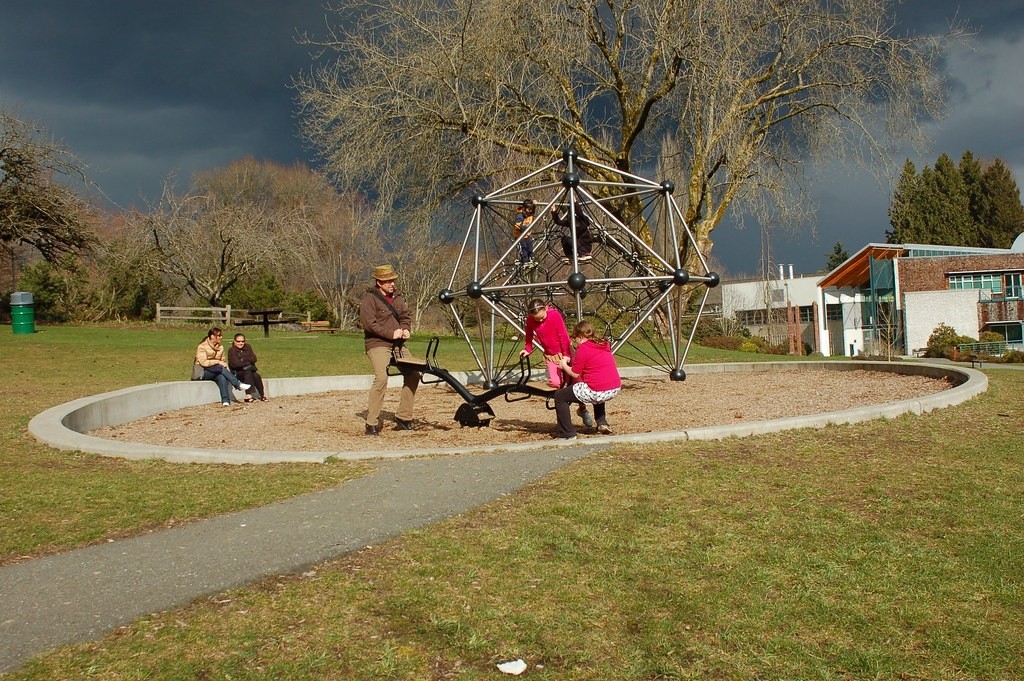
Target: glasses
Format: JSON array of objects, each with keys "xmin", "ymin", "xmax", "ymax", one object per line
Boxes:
[{"xmin": 237, "ymin": 341, "xmax": 245, "ymax": 342}]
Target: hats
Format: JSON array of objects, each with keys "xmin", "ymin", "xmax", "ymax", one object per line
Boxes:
[{"xmin": 370, "ymin": 265, "xmax": 399, "ymax": 281}]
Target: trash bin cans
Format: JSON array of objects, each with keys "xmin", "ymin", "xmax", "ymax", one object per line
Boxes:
[{"xmin": 8, "ymin": 290, "xmax": 36, "ymax": 334}]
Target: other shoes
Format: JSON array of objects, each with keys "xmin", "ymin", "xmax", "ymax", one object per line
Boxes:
[
  {"xmin": 393, "ymin": 416, "xmax": 412, "ymax": 430},
  {"xmin": 260, "ymin": 397, "xmax": 269, "ymax": 402},
  {"xmin": 239, "ymin": 383, "xmax": 251, "ymax": 392},
  {"xmin": 554, "ymin": 436, "xmax": 578, "ymax": 441},
  {"xmin": 366, "ymin": 423, "xmax": 379, "ymax": 435},
  {"xmin": 223, "ymin": 402, "xmax": 230, "ymax": 406},
  {"xmin": 577, "ymin": 408, "xmax": 593, "ymax": 427},
  {"xmin": 598, "ymin": 425, "xmax": 614, "ymax": 434},
  {"xmin": 244, "ymin": 397, "xmax": 258, "ymax": 403}
]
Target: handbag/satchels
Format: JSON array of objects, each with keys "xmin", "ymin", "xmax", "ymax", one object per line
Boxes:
[{"xmin": 191, "ymin": 364, "xmax": 205, "ymax": 380}]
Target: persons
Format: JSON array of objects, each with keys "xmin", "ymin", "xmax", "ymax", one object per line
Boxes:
[
  {"xmin": 227, "ymin": 333, "xmax": 271, "ymax": 403},
  {"xmin": 360, "ymin": 264, "xmax": 421, "ymax": 436},
  {"xmin": 520, "ymin": 299, "xmax": 592, "ymax": 427},
  {"xmin": 514, "ymin": 199, "xmax": 537, "ymax": 269},
  {"xmin": 553, "ymin": 321, "xmax": 622, "ymax": 438},
  {"xmin": 194, "ymin": 326, "xmax": 252, "ymax": 406},
  {"xmin": 551, "ymin": 195, "xmax": 592, "ymax": 265}
]
